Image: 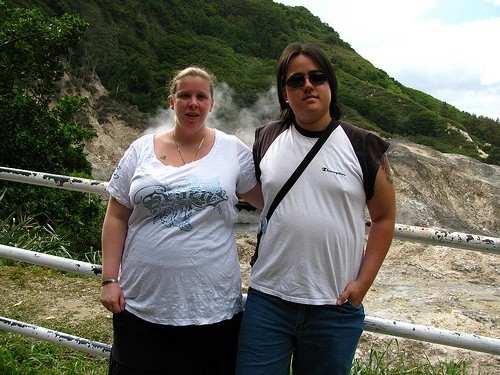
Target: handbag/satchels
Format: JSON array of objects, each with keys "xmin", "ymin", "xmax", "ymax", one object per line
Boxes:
[{"xmin": 249, "ymin": 228, "xmax": 263, "ymax": 270}]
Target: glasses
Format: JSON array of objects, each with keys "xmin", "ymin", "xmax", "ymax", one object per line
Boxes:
[{"xmin": 281, "ymin": 70, "xmax": 329, "ymax": 88}]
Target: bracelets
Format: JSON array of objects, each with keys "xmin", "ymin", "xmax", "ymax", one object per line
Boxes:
[{"xmin": 101, "ymin": 279, "xmax": 118, "ymax": 286}]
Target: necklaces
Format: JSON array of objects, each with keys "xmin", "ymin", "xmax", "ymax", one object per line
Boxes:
[{"xmin": 172, "ymin": 131, "xmax": 206, "ymax": 165}]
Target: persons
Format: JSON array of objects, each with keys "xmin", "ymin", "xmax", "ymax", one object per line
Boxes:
[
  {"xmin": 101, "ymin": 65, "xmax": 264, "ymax": 374},
  {"xmin": 240, "ymin": 42, "xmax": 396, "ymax": 375}
]
